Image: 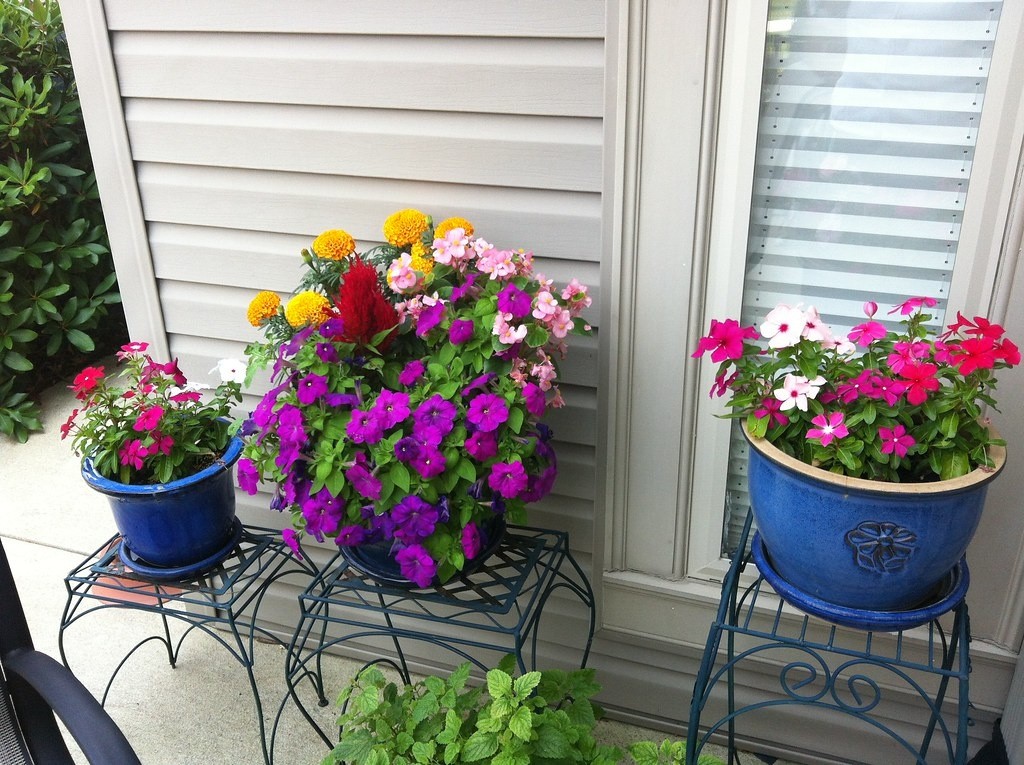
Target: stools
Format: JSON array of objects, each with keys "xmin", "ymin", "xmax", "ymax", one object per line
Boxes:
[
  {"xmin": 686, "ymin": 504, "xmax": 977, "ymax": 765},
  {"xmin": 269, "ymin": 523, "xmax": 597, "ymax": 765},
  {"xmin": 58, "ymin": 524, "xmax": 329, "ymax": 765}
]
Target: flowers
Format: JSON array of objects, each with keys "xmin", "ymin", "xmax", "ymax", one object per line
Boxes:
[
  {"xmin": 235, "ymin": 208, "xmax": 599, "ymax": 591},
  {"xmin": 60, "ymin": 335, "xmax": 248, "ymax": 484},
  {"xmin": 689, "ymin": 295, "xmax": 1022, "ymax": 483}
]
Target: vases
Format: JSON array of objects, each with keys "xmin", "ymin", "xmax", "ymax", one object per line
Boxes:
[
  {"xmin": 80, "ymin": 416, "xmax": 246, "ymax": 583},
  {"xmin": 739, "ymin": 407, "xmax": 1009, "ymax": 632},
  {"xmin": 336, "ymin": 512, "xmax": 508, "ymax": 590}
]
